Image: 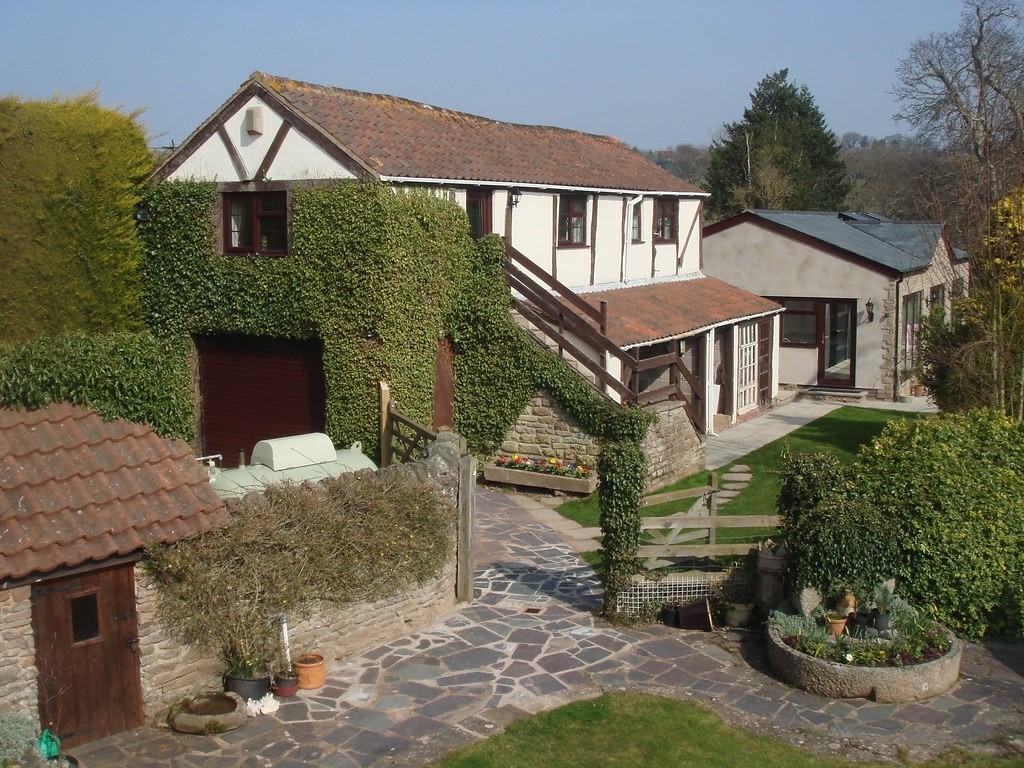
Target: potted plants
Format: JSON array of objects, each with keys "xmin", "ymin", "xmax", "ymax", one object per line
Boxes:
[
  {"xmin": 272, "ymin": 640, "xmax": 298, "ymax": 698},
  {"xmin": 293, "ymin": 634, "xmax": 325, "ymax": 690},
  {"xmin": 848, "ymin": 579, "xmax": 874, "ymax": 639},
  {"xmin": 223, "ymin": 637, "xmax": 271, "ymax": 701},
  {"xmin": 870, "ymin": 584, "xmax": 898, "ymax": 632},
  {"xmin": 824, "ymin": 611, "xmax": 848, "ymax": 635},
  {"xmin": 899, "ymin": 367, "xmax": 914, "ymax": 403}
]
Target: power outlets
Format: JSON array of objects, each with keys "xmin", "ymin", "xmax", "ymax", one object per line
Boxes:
[{"xmin": 864, "ymin": 297, "xmax": 874, "ymax": 322}]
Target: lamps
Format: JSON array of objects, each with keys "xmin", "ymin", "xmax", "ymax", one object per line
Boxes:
[
  {"xmin": 926, "ymin": 288, "xmax": 937, "ymax": 308},
  {"xmin": 508, "ymin": 187, "xmax": 522, "ymax": 208},
  {"xmin": 137, "ymin": 203, "xmax": 150, "ymax": 221},
  {"xmin": 678, "ymin": 339, "xmax": 685, "ymax": 357}
]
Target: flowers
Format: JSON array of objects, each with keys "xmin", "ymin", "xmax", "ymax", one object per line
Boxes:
[{"xmin": 495, "ymin": 452, "xmax": 592, "ymax": 479}]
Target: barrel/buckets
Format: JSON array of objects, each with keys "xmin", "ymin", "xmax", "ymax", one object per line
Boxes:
[{"xmin": 35, "ymin": 728, "xmax": 60, "ymax": 759}]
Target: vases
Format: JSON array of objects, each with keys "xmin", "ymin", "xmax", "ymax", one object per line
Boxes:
[
  {"xmin": 913, "ymin": 385, "xmax": 925, "ymax": 397},
  {"xmin": 662, "ymin": 605, "xmax": 678, "ymax": 627},
  {"xmin": 484, "ymin": 456, "xmax": 598, "ymax": 497},
  {"xmin": 677, "ymin": 597, "xmax": 714, "ymax": 632},
  {"xmin": 47, "ymin": 755, "xmax": 87, "ymax": 768},
  {"xmin": 723, "ymin": 601, "xmax": 755, "ymax": 627}
]
